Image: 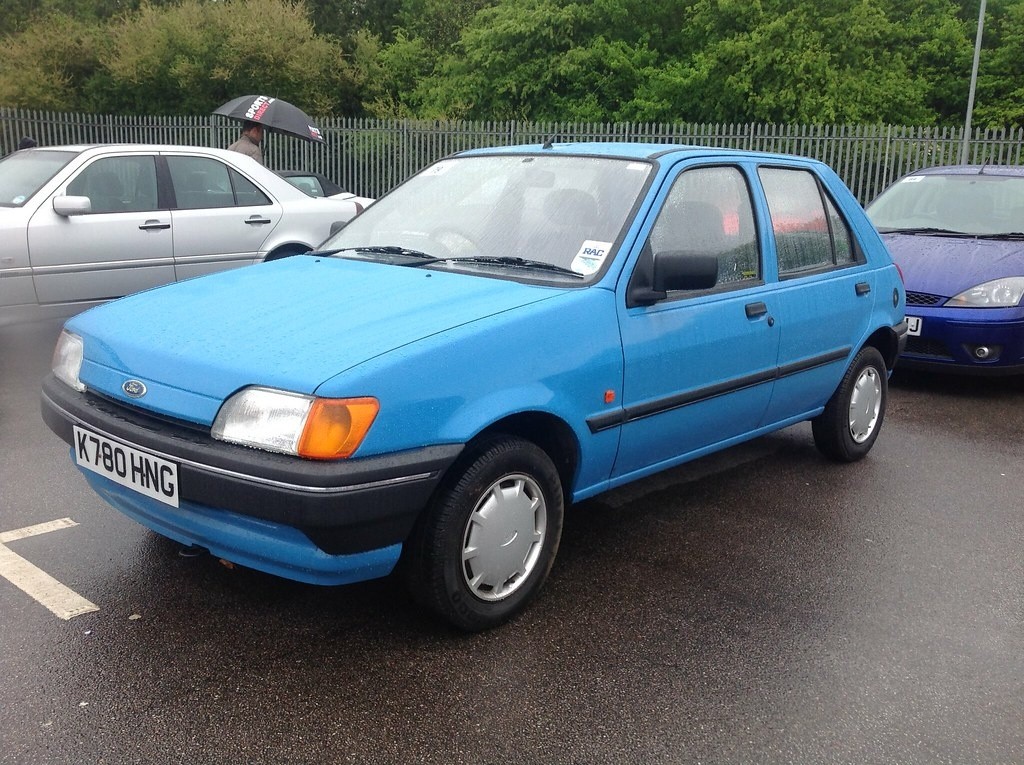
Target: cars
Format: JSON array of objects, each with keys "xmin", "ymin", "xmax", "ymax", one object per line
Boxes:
[
  {"xmin": 854, "ymin": 163, "xmax": 1024, "ymax": 378},
  {"xmin": 39, "ymin": 142, "xmax": 909, "ymax": 633},
  {"xmin": 0, "ymin": 142, "xmax": 381, "ymax": 329}
]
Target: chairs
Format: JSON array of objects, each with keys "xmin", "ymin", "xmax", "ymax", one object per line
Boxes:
[
  {"xmin": 660, "ymin": 202, "xmax": 728, "ymax": 282},
  {"xmin": 68, "ymin": 172, "xmax": 86, "ymax": 196},
  {"xmin": 91, "ymin": 172, "xmax": 125, "ymax": 213},
  {"xmin": 522, "ymin": 190, "xmax": 598, "ymax": 263}
]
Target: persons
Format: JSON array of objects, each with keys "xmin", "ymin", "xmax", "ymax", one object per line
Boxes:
[{"xmin": 226, "ymin": 120, "xmax": 264, "ymax": 165}]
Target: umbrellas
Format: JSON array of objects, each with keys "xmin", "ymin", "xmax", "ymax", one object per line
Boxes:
[{"xmin": 212, "ymin": 95, "xmax": 327, "ymax": 145}]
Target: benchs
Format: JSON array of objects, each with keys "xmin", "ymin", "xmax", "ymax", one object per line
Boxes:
[
  {"xmin": 727, "ymin": 232, "xmax": 834, "ymax": 265},
  {"xmin": 180, "ymin": 190, "xmax": 259, "ymax": 208}
]
